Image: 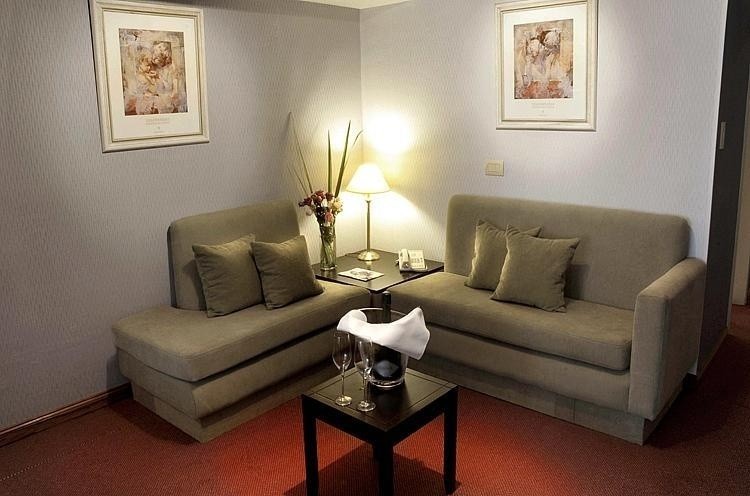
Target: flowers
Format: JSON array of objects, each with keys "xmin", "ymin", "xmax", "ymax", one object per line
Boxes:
[{"xmin": 282, "ymin": 112, "xmax": 363, "ymax": 266}]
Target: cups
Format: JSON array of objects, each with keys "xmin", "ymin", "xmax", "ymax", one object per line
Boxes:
[{"xmin": 354, "ymin": 308, "xmax": 409, "ymax": 388}]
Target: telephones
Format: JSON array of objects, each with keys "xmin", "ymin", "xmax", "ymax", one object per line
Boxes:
[{"xmin": 398, "ymin": 248, "xmax": 428, "ymax": 272}]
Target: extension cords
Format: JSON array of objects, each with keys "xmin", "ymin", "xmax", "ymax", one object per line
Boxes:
[{"xmin": 482, "ymin": 159, "xmax": 509, "ymax": 185}]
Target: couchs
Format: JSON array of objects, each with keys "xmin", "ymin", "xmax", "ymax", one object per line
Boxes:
[
  {"xmin": 389, "ymin": 193, "xmax": 705, "ymax": 444},
  {"xmin": 110, "ymin": 199, "xmax": 373, "ymax": 444}
]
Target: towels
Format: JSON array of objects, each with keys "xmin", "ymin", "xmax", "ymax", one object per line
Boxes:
[{"xmin": 336, "ymin": 304, "xmax": 431, "ymax": 363}]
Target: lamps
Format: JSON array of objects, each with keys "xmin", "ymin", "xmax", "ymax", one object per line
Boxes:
[{"xmin": 344, "ymin": 162, "xmax": 390, "ymax": 262}]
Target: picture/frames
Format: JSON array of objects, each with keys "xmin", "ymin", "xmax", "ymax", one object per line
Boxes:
[
  {"xmin": 88, "ymin": 0, "xmax": 211, "ymax": 154},
  {"xmin": 494, "ymin": 3, "xmax": 596, "ymax": 132}
]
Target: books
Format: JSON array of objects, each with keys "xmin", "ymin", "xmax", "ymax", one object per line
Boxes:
[{"xmin": 337, "ymin": 267, "xmax": 385, "ymax": 282}]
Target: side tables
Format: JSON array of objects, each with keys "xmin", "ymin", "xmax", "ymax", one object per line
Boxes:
[{"xmin": 310, "ymin": 249, "xmax": 444, "ymax": 295}]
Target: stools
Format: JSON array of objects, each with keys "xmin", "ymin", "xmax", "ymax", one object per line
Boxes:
[{"xmin": 301, "ymin": 363, "xmax": 459, "ymax": 496}]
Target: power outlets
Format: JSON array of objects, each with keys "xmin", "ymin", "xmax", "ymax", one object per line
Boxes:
[{"xmin": 485, "ymin": 160, "xmax": 504, "ymax": 176}]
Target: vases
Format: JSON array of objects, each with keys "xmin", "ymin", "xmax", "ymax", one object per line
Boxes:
[{"xmin": 320, "ymin": 226, "xmax": 336, "ymax": 270}]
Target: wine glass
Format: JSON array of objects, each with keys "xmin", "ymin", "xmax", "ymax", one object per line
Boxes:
[
  {"xmin": 355, "ymin": 337, "xmax": 376, "ymax": 412},
  {"xmin": 332, "ymin": 332, "xmax": 352, "ymax": 406}
]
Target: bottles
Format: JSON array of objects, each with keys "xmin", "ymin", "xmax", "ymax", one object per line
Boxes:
[{"xmin": 368, "ymin": 290, "xmax": 402, "ymax": 386}]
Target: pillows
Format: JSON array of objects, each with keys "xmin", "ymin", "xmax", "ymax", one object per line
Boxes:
[
  {"xmin": 250, "ymin": 236, "xmax": 325, "ymax": 309},
  {"xmin": 191, "ymin": 236, "xmax": 262, "ymax": 317},
  {"xmin": 489, "ymin": 225, "xmax": 579, "ymax": 312},
  {"xmin": 463, "ymin": 217, "xmax": 541, "ymax": 291}
]
